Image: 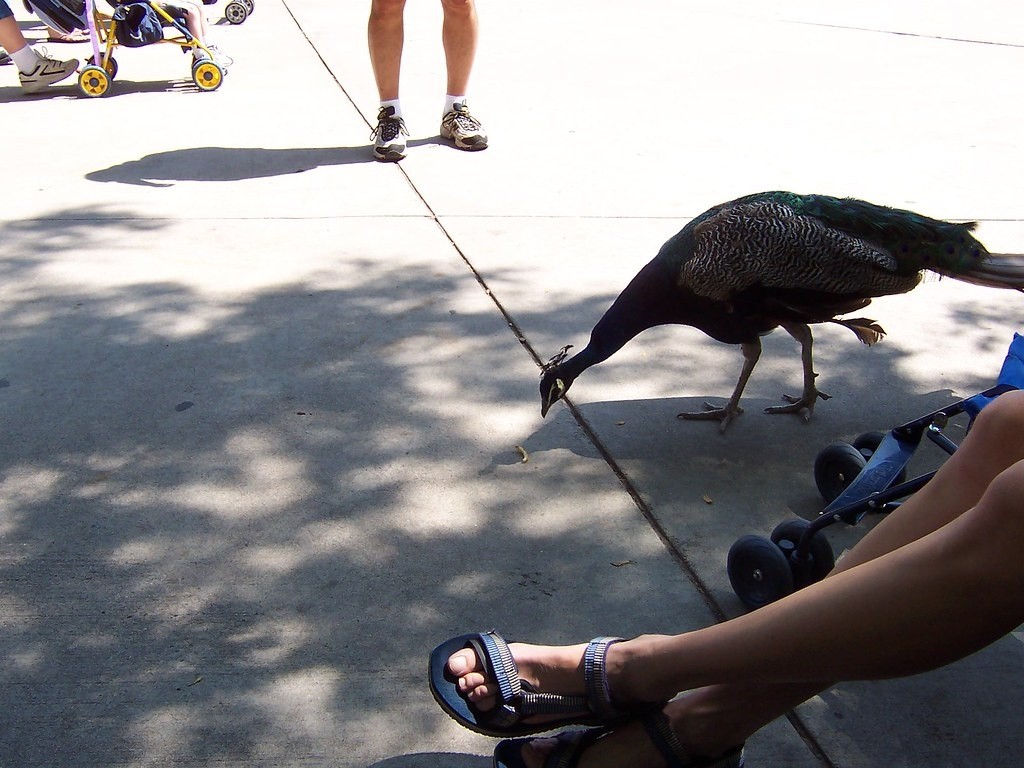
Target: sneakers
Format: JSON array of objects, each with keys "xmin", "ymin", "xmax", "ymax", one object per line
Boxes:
[
  {"xmin": 0, "ymin": 47, "xmax": 13, "ymax": 65},
  {"xmin": 370, "ymin": 106, "xmax": 410, "ymax": 162},
  {"xmin": 440, "ymin": 100, "xmax": 488, "ymax": 151},
  {"xmin": 19, "ymin": 46, "xmax": 79, "ymax": 94}
]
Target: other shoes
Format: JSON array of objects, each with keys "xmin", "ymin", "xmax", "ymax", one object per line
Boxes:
[{"xmin": 192, "ymin": 41, "xmax": 234, "ymax": 66}]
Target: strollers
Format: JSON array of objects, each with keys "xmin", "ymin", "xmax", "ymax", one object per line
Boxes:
[
  {"xmin": 202, "ymin": 0, "xmax": 255, "ymax": 25},
  {"xmin": 21, "ymin": 0, "xmax": 234, "ymax": 101},
  {"xmin": 729, "ymin": 336, "xmax": 1024, "ymax": 601}
]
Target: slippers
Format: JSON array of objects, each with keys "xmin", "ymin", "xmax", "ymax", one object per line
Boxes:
[{"xmin": 47, "ymin": 27, "xmax": 91, "ymax": 43}]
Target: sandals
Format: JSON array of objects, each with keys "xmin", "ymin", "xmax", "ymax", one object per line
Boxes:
[
  {"xmin": 494, "ymin": 700, "xmax": 745, "ymax": 768},
  {"xmin": 429, "ymin": 628, "xmax": 674, "ymax": 738}
]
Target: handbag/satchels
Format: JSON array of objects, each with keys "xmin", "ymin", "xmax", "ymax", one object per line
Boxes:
[{"xmin": 112, "ymin": 2, "xmax": 163, "ymax": 48}]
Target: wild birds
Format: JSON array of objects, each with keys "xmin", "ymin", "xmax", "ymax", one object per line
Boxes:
[{"xmin": 541, "ymin": 191, "xmax": 1024, "ymax": 429}]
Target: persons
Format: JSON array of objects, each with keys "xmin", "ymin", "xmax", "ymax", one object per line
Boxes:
[
  {"xmin": 428, "ymin": 391, "xmax": 1024, "ymax": 768},
  {"xmin": 0, "ymin": 0, "xmax": 488, "ymax": 162}
]
